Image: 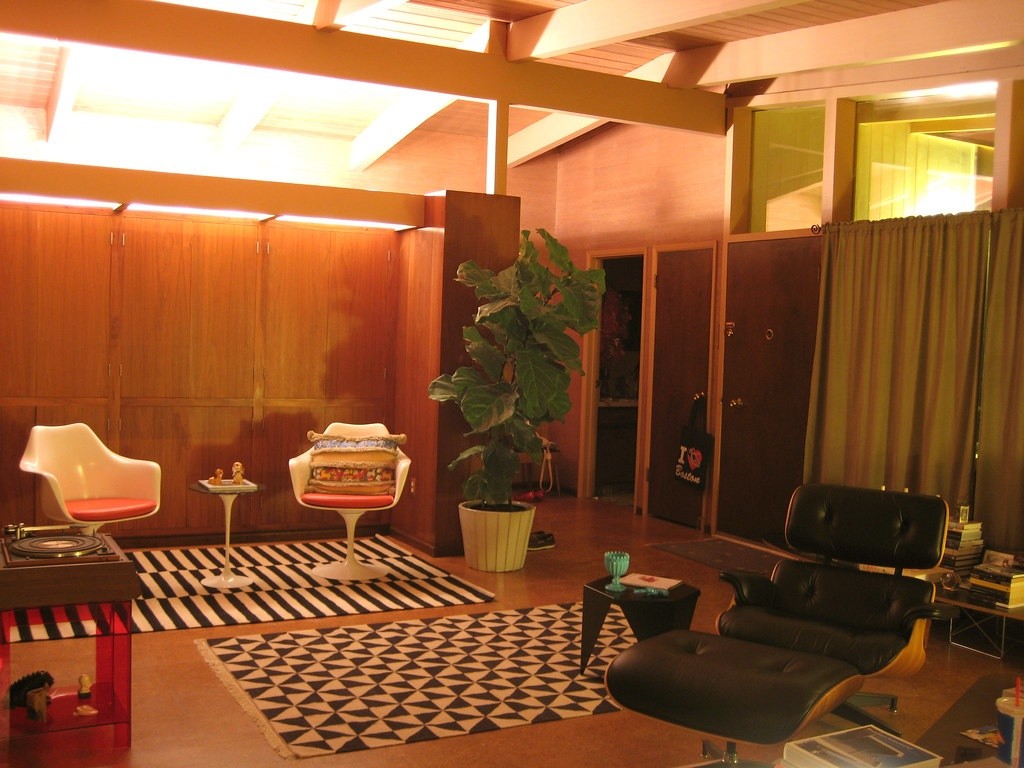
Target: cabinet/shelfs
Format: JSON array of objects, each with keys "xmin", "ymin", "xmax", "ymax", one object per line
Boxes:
[{"xmin": 0, "ymin": 157, "xmax": 522, "ymax": 558}]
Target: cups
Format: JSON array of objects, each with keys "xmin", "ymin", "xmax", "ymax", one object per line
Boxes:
[{"xmin": 995, "ymin": 697, "xmax": 1024, "ymax": 768}]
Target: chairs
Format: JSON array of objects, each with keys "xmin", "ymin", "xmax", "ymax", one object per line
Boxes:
[
  {"xmin": 604, "ymin": 481, "xmax": 962, "ymax": 768},
  {"xmin": 289, "ymin": 422, "xmax": 411, "ymax": 580},
  {"xmin": 511, "ymin": 424, "xmax": 562, "ymax": 500},
  {"xmin": 19, "ymin": 423, "xmax": 161, "ymax": 537}
]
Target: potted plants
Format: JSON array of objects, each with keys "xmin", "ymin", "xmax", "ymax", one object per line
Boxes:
[{"xmin": 429, "ymin": 228, "xmax": 606, "ymax": 573}]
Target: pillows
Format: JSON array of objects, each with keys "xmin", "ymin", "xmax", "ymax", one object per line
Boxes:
[{"xmin": 304, "ymin": 430, "xmax": 407, "ymax": 498}]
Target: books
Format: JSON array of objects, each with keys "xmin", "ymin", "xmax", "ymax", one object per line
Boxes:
[
  {"xmin": 939, "ymin": 518, "xmax": 1024, "ymax": 609},
  {"xmin": 783, "ymin": 724, "xmax": 943, "ymax": 768}
]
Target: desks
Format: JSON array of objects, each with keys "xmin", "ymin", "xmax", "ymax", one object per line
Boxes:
[
  {"xmin": 187, "ymin": 482, "xmax": 268, "ymax": 589},
  {"xmin": 579, "ymin": 574, "xmax": 701, "ymax": 675},
  {"xmin": 932, "ymin": 582, "xmax": 1024, "ymax": 659}
]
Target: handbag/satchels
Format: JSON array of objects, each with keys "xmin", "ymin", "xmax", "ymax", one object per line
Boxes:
[{"xmin": 671, "ymin": 396, "xmax": 713, "ymax": 492}]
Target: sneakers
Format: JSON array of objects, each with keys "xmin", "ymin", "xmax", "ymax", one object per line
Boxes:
[{"xmin": 526, "ymin": 530, "xmax": 555, "ymax": 550}]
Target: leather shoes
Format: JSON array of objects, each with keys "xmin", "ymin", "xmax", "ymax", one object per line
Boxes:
[{"xmin": 515, "ymin": 490, "xmax": 543, "ymax": 504}]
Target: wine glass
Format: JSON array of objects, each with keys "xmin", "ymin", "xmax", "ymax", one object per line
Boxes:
[{"xmin": 606, "ymin": 552, "xmax": 629, "ymax": 592}]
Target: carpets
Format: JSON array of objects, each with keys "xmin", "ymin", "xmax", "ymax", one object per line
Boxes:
[
  {"xmin": 0, "ymin": 534, "xmax": 497, "ymax": 644},
  {"xmin": 193, "ymin": 600, "xmax": 637, "ymax": 761}
]
